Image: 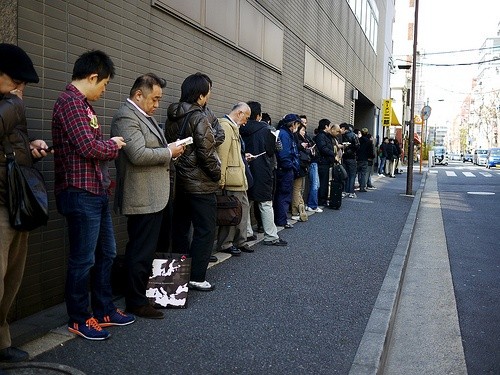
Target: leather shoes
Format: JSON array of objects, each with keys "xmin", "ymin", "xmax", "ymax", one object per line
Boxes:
[
  {"xmin": 0, "ymin": 347, "xmax": 29, "ymax": 363},
  {"xmin": 218, "ymin": 245, "xmax": 241, "ymax": 255},
  {"xmin": 240, "ymin": 244, "xmax": 255, "ymax": 252}
]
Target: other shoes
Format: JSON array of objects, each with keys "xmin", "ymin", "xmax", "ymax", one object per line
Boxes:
[
  {"xmin": 247, "ymin": 235, "xmax": 257, "ymax": 242},
  {"xmin": 264, "ymin": 238, "xmax": 288, "ymax": 246},
  {"xmin": 126, "ymin": 303, "xmax": 163, "ymax": 319}
]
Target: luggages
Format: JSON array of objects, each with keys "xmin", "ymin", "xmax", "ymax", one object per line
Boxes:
[{"xmin": 328, "ymin": 162, "xmax": 343, "ymax": 210}]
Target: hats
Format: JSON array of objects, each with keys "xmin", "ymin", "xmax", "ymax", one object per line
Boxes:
[
  {"xmin": 0, "ymin": 43, "xmax": 39, "ymax": 84},
  {"xmin": 286, "ymin": 114, "xmax": 303, "ymax": 122}
]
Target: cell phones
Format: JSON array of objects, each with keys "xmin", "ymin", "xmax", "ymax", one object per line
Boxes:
[
  {"xmin": 249, "ymin": 150, "xmax": 266, "ymax": 158},
  {"xmin": 46, "ymin": 143, "xmax": 63, "ymax": 152},
  {"xmin": 304, "ymin": 138, "xmax": 316, "ymax": 149},
  {"xmin": 123, "ymin": 139, "xmax": 132, "ymax": 143}
]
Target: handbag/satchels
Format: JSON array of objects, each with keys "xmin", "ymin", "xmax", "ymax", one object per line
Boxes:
[
  {"xmin": 215, "ymin": 186, "xmax": 242, "ymax": 226},
  {"xmin": 333, "ymin": 162, "xmax": 348, "ymax": 181},
  {"xmin": 141, "ymin": 251, "xmax": 193, "ymax": 308},
  {"xmin": 7, "ymin": 158, "xmax": 49, "ymax": 230}
]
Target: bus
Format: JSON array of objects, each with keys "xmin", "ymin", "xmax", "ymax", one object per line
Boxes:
[{"xmin": 431, "ymin": 146, "xmax": 448, "ymax": 166}]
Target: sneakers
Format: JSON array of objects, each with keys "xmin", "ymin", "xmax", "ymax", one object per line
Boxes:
[
  {"xmin": 67, "ymin": 314, "xmax": 111, "ymax": 341},
  {"xmin": 283, "ymin": 172, "xmax": 403, "ymax": 228},
  {"xmin": 188, "ymin": 280, "xmax": 214, "ymax": 291},
  {"xmin": 95, "ymin": 306, "xmax": 135, "ymax": 328}
]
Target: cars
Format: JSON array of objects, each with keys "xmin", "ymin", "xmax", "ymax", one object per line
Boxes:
[
  {"xmin": 463, "ymin": 154, "xmax": 473, "ymax": 163},
  {"xmin": 473, "ymin": 149, "xmax": 489, "ymax": 166},
  {"xmin": 486, "ymin": 147, "xmax": 500, "ymax": 169}
]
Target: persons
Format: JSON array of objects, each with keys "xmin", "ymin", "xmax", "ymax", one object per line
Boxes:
[
  {"xmin": 333, "ymin": 122, "xmax": 350, "ymax": 198},
  {"xmin": 273, "ymin": 113, "xmax": 301, "ymax": 230},
  {"xmin": 254, "ymin": 111, "xmax": 281, "ymax": 234},
  {"xmin": 364, "ymin": 134, "xmax": 378, "ymax": 190},
  {"xmin": 289, "ymin": 114, "xmax": 306, "ymax": 224},
  {"xmin": 351, "ymin": 128, "xmax": 373, "ymax": 191},
  {"xmin": 107, "ymin": 71, "xmax": 187, "ymax": 321},
  {"xmin": 358, "ymin": 126, "xmax": 373, "ymax": 193},
  {"xmin": 164, "ymin": 74, "xmax": 225, "ymax": 295},
  {"xmin": 295, "ymin": 124, "xmax": 314, "ymax": 217},
  {"xmin": 242, "ymin": 99, "xmax": 289, "ymax": 248},
  {"xmin": 373, "ymin": 136, "xmax": 404, "ymax": 179},
  {"xmin": 209, "ymin": 102, "xmax": 257, "ymax": 257},
  {"xmin": 51, "ymin": 49, "xmax": 138, "ymax": 342},
  {"xmin": 325, "ymin": 121, "xmax": 348, "ymax": 204},
  {"xmin": 187, "ymin": 70, "xmax": 228, "ymax": 264},
  {"xmin": 312, "ymin": 116, "xmax": 339, "ymax": 208},
  {"xmin": 341, "ymin": 122, "xmax": 360, "ymax": 198},
  {"xmin": 298, "ymin": 114, "xmax": 324, "ymax": 215},
  {"xmin": 312, "ymin": 124, "xmax": 331, "ymax": 205},
  {"xmin": 0, "ymin": 40, "xmax": 54, "ymax": 361}
]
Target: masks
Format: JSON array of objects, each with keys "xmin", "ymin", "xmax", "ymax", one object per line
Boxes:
[{"xmin": 292, "ymin": 126, "xmax": 298, "ymax": 133}]
